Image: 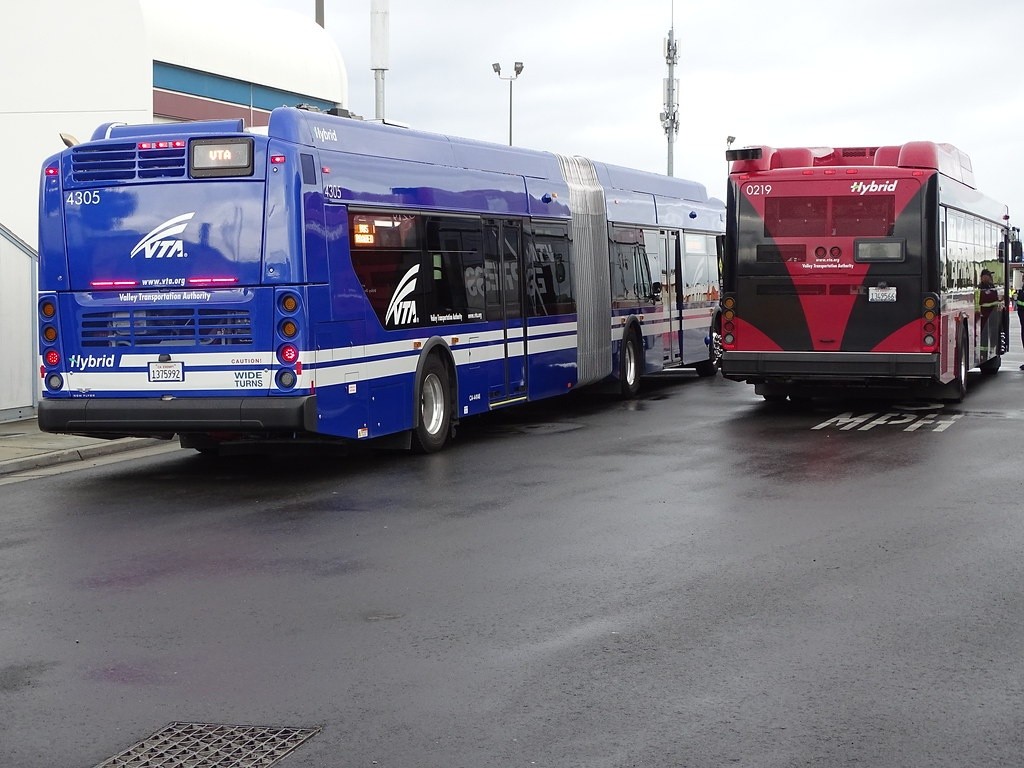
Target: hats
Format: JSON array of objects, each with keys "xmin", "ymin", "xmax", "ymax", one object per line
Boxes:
[
  {"xmin": 981, "ymin": 269, "xmax": 995, "ymax": 275},
  {"xmin": 1019, "ymin": 270, "xmax": 1024, "ymax": 274}
]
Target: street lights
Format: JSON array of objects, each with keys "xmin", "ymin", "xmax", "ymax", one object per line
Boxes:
[{"xmin": 493, "ymin": 62, "xmax": 524, "ymax": 146}]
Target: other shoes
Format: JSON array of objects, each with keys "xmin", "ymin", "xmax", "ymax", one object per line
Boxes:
[{"xmin": 1019, "ymin": 365, "xmax": 1024, "ymax": 370}]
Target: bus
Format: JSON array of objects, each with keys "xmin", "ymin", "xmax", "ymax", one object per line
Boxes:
[
  {"xmin": 37, "ymin": 103, "xmax": 725, "ymax": 454},
  {"xmin": 720, "ymin": 140, "xmax": 1022, "ymax": 408}
]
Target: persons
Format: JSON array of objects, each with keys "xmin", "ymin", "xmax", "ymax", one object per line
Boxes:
[
  {"xmin": 973, "ymin": 269, "xmax": 999, "ymax": 355},
  {"xmin": 1016, "ymin": 270, "xmax": 1024, "ymax": 370},
  {"xmin": 1009, "ymin": 286, "xmax": 1015, "ymax": 308}
]
